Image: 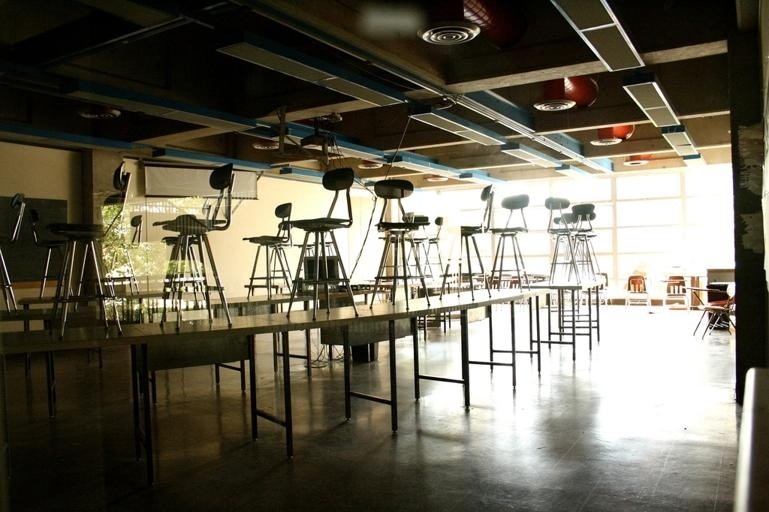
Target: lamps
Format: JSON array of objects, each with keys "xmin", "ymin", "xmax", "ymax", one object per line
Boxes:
[
  {"xmin": 621, "ymin": 155, "xmax": 651, "ymax": 166},
  {"xmin": 409, "ymin": 0, "xmax": 530, "ymax": 54},
  {"xmin": 77, "ymin": 106, "xmax": 120, "ymax": 120},
  {"xmin": 250, "ymin": 136, "xmax": 280, "ymax": 151},
  {"xmin": 509, "ymin": 75, "xmax": 600, "ymax": 113},
  {"xmin": 580, "ymin": 125, "xmax": 635, "ymax": 146}
]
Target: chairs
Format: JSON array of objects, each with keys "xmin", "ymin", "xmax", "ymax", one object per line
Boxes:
[
  {"xmin": 626, "ymin": 276, "xmax": 651, "ymax": 308},
  {"xmin": 663, "ymin": 276, "xmax": 689, "ymax": 310},
  {"xmin": 681, "ymin": 286, "xmax": 735, "ymax": 340}
]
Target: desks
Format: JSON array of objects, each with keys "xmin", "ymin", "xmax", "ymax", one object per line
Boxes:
[{"xmin": 706, "ymin": 269, "xmax": 736, "ymax": 331}]
